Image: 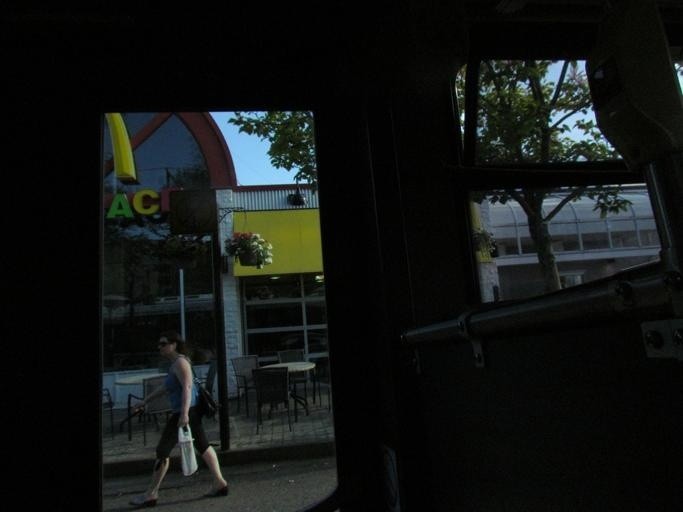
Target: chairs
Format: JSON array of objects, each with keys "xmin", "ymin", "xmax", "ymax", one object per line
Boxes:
[
  {"xmin": 102, "ymin": 387, "xmax": 115, "ymax": 441},
  {"xmin": 229, "ymin": 353, "xmax": 259, "ymax": 416},
  {"xmin": 156, "ymin": 359, "xmax": 172, "ymax": 373},
  {"xmin": 277, "ymin": 348, "xmax": 309, "ymax": 405},
  {"xmin": 126, "ymin": 374, "xmax": 172, "ymax": 448},
  {"xmin": 249, "ymin": 366, "xmax": 293, "ymax": 434},
  {"xmin": 312, "ymin": 347, "xmax": 331, "ymax": 409},
  {"xmin": 193, "ymin": 360, "xmax": 218, "ymax": 423}
]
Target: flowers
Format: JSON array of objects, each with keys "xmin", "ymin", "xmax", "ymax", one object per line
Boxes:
[
  {"xmin": 222, "ymin": 227, "xmax": 274, "ymax": 269},
  {"xmin": 473, "ymin": 225, "xmax": 497, "ymax": 259}
]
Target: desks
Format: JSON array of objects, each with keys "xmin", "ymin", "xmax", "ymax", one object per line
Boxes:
[
  {"xmin": 112, "ymin": 373, "xmax": 168, "ymax": 430},
  {"xmin": 261, "ymin": 361, "xmax": 316, "ymax": 416}
]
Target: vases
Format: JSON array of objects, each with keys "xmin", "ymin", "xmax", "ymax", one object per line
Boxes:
[
  {"xmin": 474, "ymin": 242, "xmax": 480, "ymax": 252},
  {"xmin": 236, "ymin": 248, "xmax": 258, "ymax": 266}
]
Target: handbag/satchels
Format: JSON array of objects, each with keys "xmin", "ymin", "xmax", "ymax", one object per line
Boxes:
[{"xmin": 198, "ymin": 384, "xmax": 218, "ymax": 417}]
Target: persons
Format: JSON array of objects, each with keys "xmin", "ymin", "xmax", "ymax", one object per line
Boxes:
[{"xmin": 130, "ymin": 333, "xmax": 228, "ymax": 506}]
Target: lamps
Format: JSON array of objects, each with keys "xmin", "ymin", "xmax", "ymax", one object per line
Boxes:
[{"xmin": 286, "ymin": 186, "xmax": 308, "ymax": 207}]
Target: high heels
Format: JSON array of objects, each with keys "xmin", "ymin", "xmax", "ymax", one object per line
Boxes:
[
  {"xmin": 203, "ymin": 485, "xmax": 228, "ymax": 497},
  {"xmin": 129, "ymin": 495, "xmax": 156, "ymax": 507}
]
